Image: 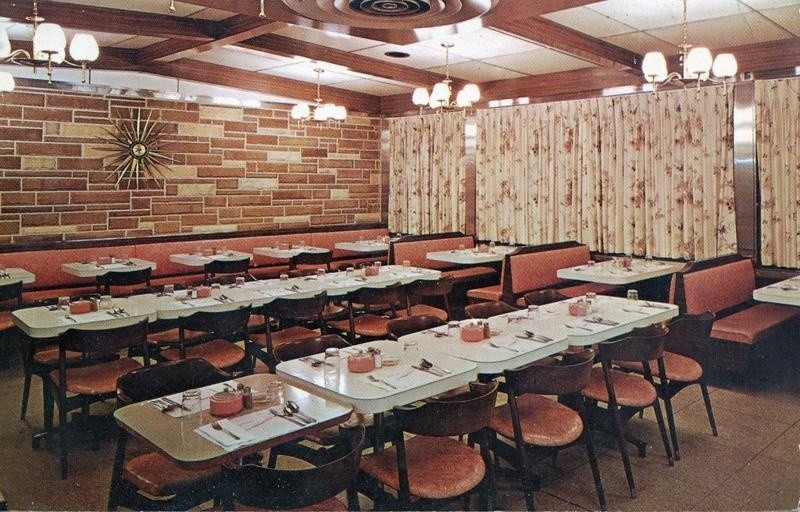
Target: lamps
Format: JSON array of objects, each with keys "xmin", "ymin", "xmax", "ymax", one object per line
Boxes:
[
  {"xmin": 410, "ymin": 42, "xmax": 483, "ymax": 123},
  {"xmin": 289, "ymin": 66, "xmax": 349, "ymax": 125},
  {"xmin": 636, "ymin": 0, "xmax": 739, "ymax": 103},
  {"xmin": 0, "ymin": 0, "xmax": 102, "ymax": 99}
]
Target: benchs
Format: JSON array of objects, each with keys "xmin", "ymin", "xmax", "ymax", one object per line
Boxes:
[{"xmin": 681, "ymin": 252, "xmax": 798, "ymax": 379}]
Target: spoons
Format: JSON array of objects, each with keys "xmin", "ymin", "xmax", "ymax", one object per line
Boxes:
[
  {"xmin": 289, "ymin": 400, "xmax": 317, "ymax": 424},
  {"xmin": 523, "ymin": 329, "xmax": 555, "ymax": 342},
  {"xmin": 419, "ymin": 358, "xmax": 450, "ymax": 376},
  {"xmin": 149, "ymin": 400, "xmax": 177, "ymax": 413},
  {"xmin": 299, "ymin": 356, "xmax": 321, "ymax": 368}
]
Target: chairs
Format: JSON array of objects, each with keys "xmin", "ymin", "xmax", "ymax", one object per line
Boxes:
[{"xmin": 1, "ymin": 219, "xmax": 717, "ymax": 512}]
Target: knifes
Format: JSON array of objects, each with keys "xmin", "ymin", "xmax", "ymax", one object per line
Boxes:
[
  {"xmin": 516, "ymin": 335, "xmax": 547, "ymax": 344},
  {"xmin": 411, "ymin": 364, "xmax": 441, "ymax": 377},
  {"xmin": 162, "ymin": 397, "xmax": 192, "ymax": 413},
  {"xmin": 267, "ymin": 408, "xmax": 306, "ymax": 429},
  {"xmin": 563, "ymin": 323, "xmax": 593, "ymax": 333}
]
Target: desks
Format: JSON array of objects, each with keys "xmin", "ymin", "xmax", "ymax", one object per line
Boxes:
[{"xmin": 753, "ymin": 271, "xmax": 800, "ymax": 305}]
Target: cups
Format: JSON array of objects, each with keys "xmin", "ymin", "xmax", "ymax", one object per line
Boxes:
[
  {"xmin": 52, "ymin": 232, "xmax": 496, "ymax": 319},
  {"xmin": 181, "ymin": 390, "xmax": 202, "ymax": 421},
  {"xmin": 526, "ymin": 254, "xmax": 653, "ymax": 326},
  {"xmin": 403, "ymin": 339, "xmax": 420, "ymax": 363},
  {"xmin": 267, "ymin": 382, "xmax": 287, "ymax": 407},
  {"xmin": 324, "ymin": 347, "xmax": 340, "ymax": 377},
  {"xmin": 447, "ymin": 320, "xmax": 462, "ymax": 346}
]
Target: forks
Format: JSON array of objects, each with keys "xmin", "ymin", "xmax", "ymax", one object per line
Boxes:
[
  {"xmin": 485, "ymin": 342, "xmax": 521, "ymax": 354},
  {"xmin": 209, "ymin": 421, "xmax": 241, "ymax": 443},
  {"xmin": 365, "ymin": 374, "xmax": 398, "ymax": 394}
]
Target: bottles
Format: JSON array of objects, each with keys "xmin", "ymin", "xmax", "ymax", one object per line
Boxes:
[
  {"xmin": 478, "ymin": 320, "xmax": 491, "ymax": 339},
  {"xmin": 236, "ymin": 383, "xmax": 253, "ymax": 409},
  {"xmin": 367, "ymin": 347, "xmax": 382, "ymax": 369}
]
